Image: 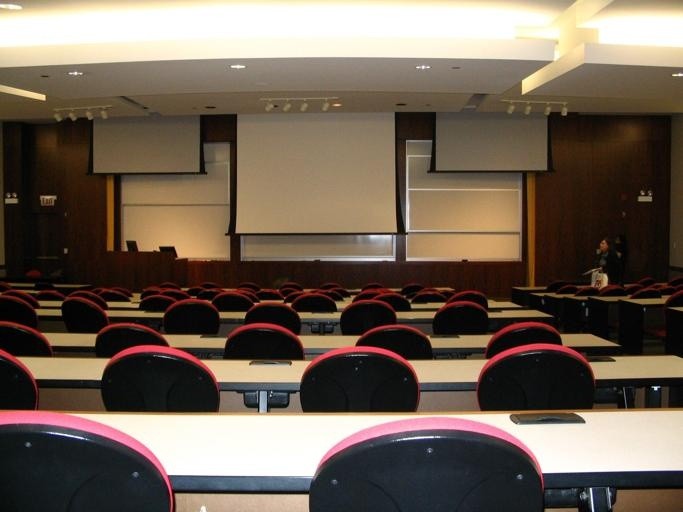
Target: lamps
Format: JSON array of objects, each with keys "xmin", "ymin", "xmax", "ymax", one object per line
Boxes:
[
  {"xmin": 53, "ymin": 105, "xmax": 114, "ymax": 124},
  {"xmin": 500, "ymin": 100, "xmax": 568, "ymax": 118},
  {"xmin": 257, "ymin": 98, "xmax": 338, "ymax": 113}
]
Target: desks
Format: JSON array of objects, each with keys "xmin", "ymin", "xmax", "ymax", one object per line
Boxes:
[{"xmin": 102, "ymin": 251, "xmax": 188, "ymax": 298}]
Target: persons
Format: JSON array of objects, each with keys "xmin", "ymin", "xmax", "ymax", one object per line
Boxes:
[{"xmin": 592, "ymin": 239, "xmax": 613, "ymax": 277}]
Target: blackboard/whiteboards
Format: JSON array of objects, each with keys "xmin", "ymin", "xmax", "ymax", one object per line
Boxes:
[
  {"xmin": 119, "ymin": 205, "xmax": 231, "ymax": 261},
  {"xmin": 240, "ymin": 235, "xmax": 397, "ymax": 262},
  {"xmin": 120, "ymin": 141, "xmax": 230, "ymax": 204},
  {"xmin": 405, "ymin": 139, "xmax": 523, "ymax": 263}
]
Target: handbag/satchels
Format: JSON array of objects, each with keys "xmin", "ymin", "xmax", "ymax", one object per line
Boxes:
[{"xmin": 591, "ymin": 270, "xmax": 608, "ymax": 290}]
